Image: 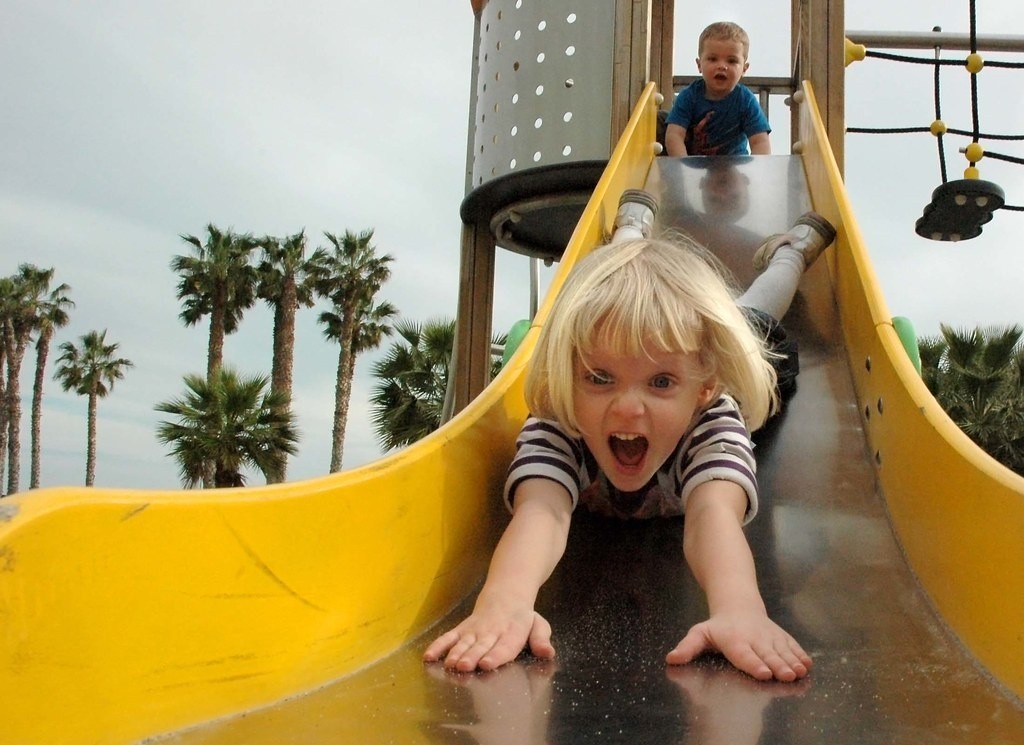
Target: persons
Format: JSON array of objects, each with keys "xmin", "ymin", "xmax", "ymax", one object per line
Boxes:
[
  {"xmin": 422, "ymin": 189, "xmax": 837, "ymax": 682},
  {"xmin": 656, "ymin": 22, "xmax": 772, "ymax": 157}
]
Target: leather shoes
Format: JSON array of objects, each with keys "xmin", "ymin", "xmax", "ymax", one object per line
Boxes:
[
  {"xmin": 752, "ymin": 210, "xmax": 837, "ymax": 276},
  {"xmin": 609, "ymin": 188, "xmax": 659, "ymax": 238}
]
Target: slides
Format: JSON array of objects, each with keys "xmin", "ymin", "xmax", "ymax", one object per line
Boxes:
[{"xmin": 0, "ymin": 78, "xmax": 1024, "ymax": 745}]
